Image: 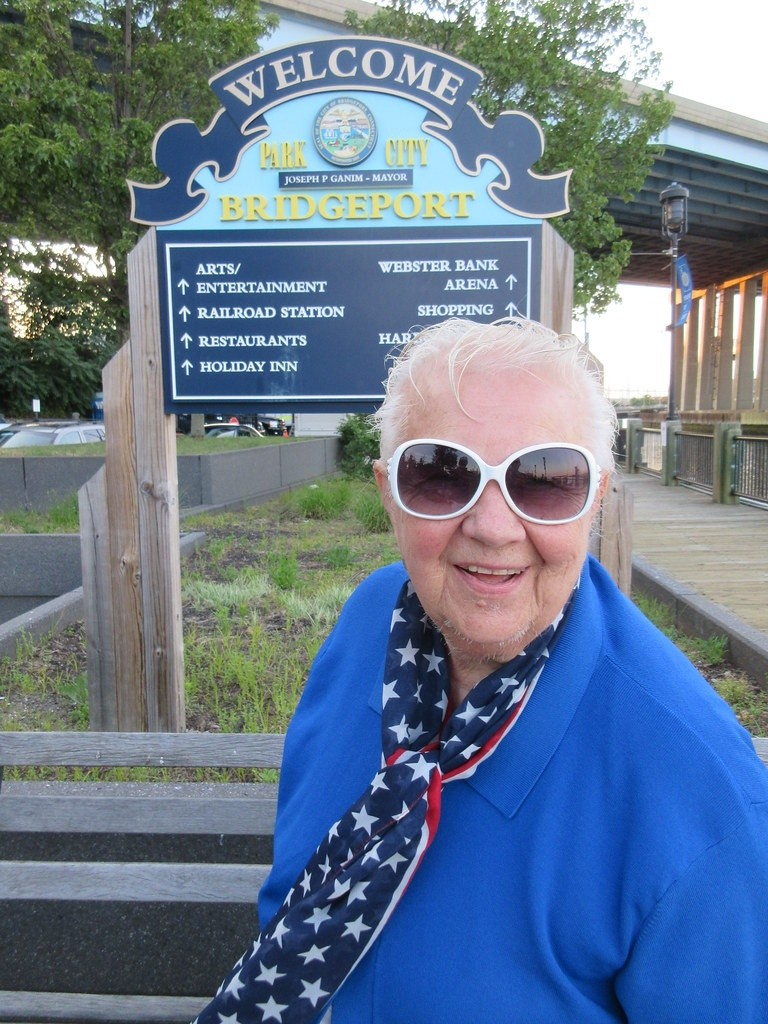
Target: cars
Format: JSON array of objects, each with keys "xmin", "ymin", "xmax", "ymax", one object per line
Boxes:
[{"xmin": 0, "ymin": 411, "xmax": 290, "ymax": 447}]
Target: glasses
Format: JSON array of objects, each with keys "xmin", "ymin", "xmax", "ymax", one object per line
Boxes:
[{"xmin": 387, "ymin": 439, "xmax": 601, "ymax": 526}]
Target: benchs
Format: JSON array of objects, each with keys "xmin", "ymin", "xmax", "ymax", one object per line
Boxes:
[{"xmin": 0, "ymin": 731, "xmax": 286, "ymax": 1024}]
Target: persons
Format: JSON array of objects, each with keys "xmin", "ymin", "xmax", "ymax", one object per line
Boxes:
[{"xmin": 195, "ymin": 317, "xmax": 768, "ymax": 1024}]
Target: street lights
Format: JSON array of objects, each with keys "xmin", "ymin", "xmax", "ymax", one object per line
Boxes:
[{"xmin": 659, "ymin": 182, "xmax": 691, "ymax": 421}]
